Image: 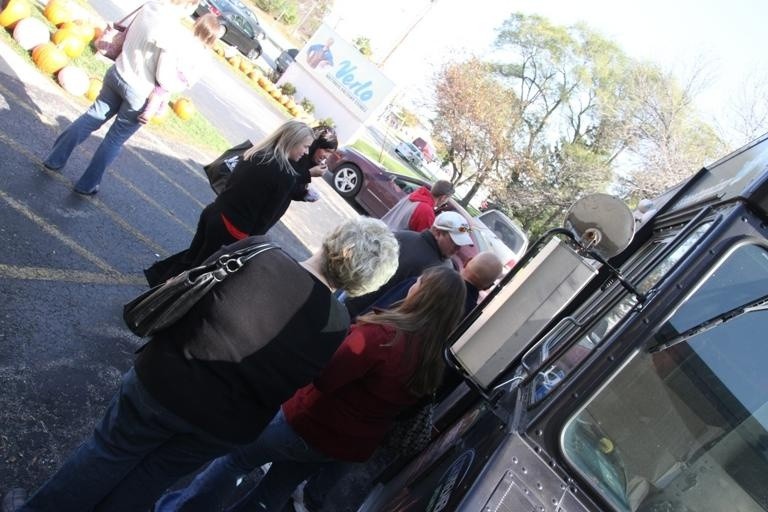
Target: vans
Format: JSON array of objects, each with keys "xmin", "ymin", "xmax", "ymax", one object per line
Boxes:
[{"xmin": 414, "ymin": 138, "xmax": 433, "ymax": 164}]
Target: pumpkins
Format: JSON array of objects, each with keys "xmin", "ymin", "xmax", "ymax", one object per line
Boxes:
[
  {"xmin": 173, "ymin": 96, "xmax": 195, "ymax": 121},
  {"xmin": 215, "ymin": 46, "xmax": 305, "ymax": 117},
  {"xmin": 0, "ymin": 0, "xmax": 103, "ymax": 102}
]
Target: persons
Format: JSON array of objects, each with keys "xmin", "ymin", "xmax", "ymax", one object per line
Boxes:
[
  {"xmin": 133, "ymin": 11, "xmax": 221, "ymax": 123},
  {"xmin": 38, "ymin": 0, "xmax": 201, "ymax": 197},
  {"xmin": 304, "ymin": 38, "xmax": 334, "ymax": 69},
  {"xmin": 0, "ymin": 119, "xmax": 502, "ymax": 512}
]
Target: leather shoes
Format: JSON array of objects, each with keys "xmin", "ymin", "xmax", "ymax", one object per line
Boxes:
[
  {"xmin": 73, "ymin": 184, "xmax": 100, "ymax": 194},
  {"xmin": 45, "ymin": 159, "xmax": 69, "ymax": 169}
]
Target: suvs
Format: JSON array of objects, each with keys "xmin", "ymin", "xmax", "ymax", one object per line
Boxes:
[{"xmin": 342, "ymin": 132, "xmax": 768, "ymax": 512}]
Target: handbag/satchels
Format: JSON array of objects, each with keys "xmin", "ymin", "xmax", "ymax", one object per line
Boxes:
[
  {"xmin": 94, "ymin": 0, "xmax": 153, "ymax": 61},
  {"xmin": 364, "ymin": 389, "xmax": 434, "ymax": 478},
  {"xmin": 204, "ymin": 139, "xmax": 254, "ymax": 196},
  {"xmin": 123, "ymin": 242, "xmax": 282, "ymax": 338}
]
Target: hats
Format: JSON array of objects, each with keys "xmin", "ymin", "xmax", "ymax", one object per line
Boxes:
[{"xmin": 433, "ymin": 211, "xmax": 474, "ymax": 247}]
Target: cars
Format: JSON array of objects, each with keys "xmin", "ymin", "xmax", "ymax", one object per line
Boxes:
[
  {"xmin": 472, "ymin": 210, "xmax": 528, "ymax": 282},
  {"xmin": 395, "ymin": 141, "xmax": 423, "ymax": 166},
  {"xmin": 194, "ymin": 0, "xmax": 266, "ymax": 60},
  {"xmin": 275, "ymin": 49, "xmax": 298, "ymax": 71},
  {"xmin": 323, "ymin": 147, "xmax": 486, "ymax": 266}
]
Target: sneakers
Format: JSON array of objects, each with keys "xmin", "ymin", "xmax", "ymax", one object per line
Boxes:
[
  {"xmin": 155, "ymin": 111, "xmax": 166, "ymax": 117},
  {"xmin": 136, "ymin": 113, "xmax": 150, "ymax": 124},
  {"xmin": 2, "ymin": 488, "xmax": 28, "ymax": 512},
  {"xmin": 291, "ymin": 480, "xmax": 310, "ymax": 512}
]
